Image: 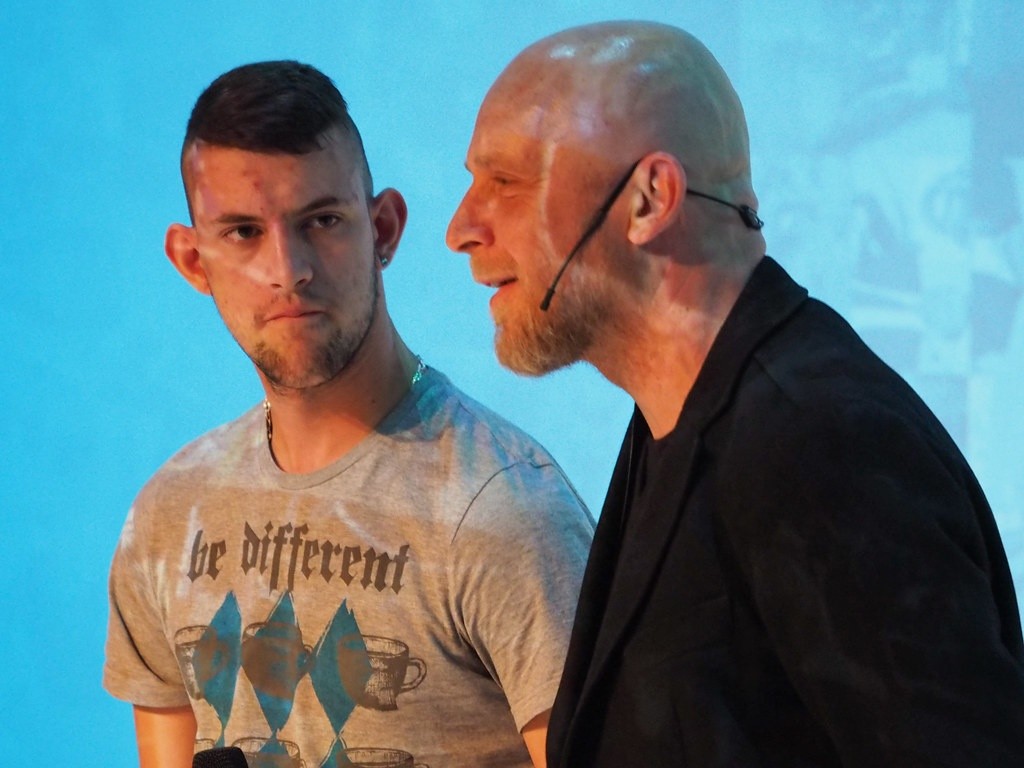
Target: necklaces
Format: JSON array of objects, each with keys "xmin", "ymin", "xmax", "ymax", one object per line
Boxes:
[{"xmin": 264, "ymin": 356, "xmax": 427, "ymax": 439}]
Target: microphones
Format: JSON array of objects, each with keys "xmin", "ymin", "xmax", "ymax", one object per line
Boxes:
[
  {"xmin": 194, "ymin": 747, "xmax": 249, "ymax": 768},
  {"xmin": 541, "ymin": 165, "xmax": 638, "ymax": 311}
]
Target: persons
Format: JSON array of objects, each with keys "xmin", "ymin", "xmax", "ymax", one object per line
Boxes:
[
  {"xmin": 104, "ymin": 61, "xmax": 597, "ymax": 768},
  {"xmin": 445, "ymin": 20, "xmax": 1024, "ymax": 768}
]
